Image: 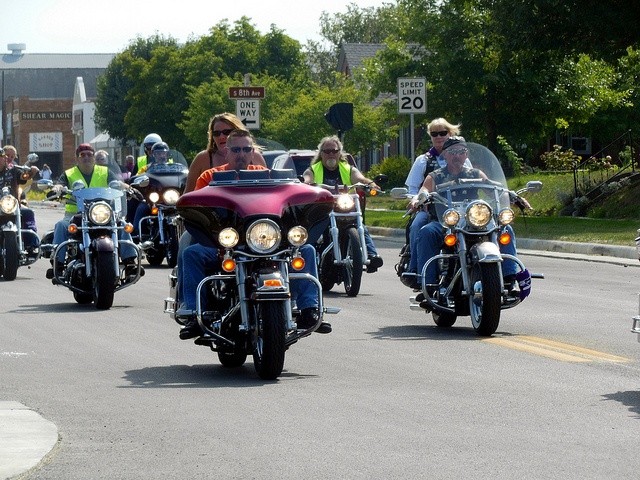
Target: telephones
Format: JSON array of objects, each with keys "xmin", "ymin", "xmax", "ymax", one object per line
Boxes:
[
  {"xmin": 399, "ymin": 78, "xmax": 426, "ymax": 113},
  {"xmin": 229, "ymin": 87, "xmax": 264, "ymax": 98},
  {"xmin": 237, "ymin": 100, "xmax": 260, "ymax": 130}
]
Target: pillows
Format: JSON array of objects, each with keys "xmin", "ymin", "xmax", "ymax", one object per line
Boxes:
[
  {"xmin": 151, "ymin": 141, "xmax": 170, "ymax": 157},
  {"xmin": 143, "ymin": 134, "xmax": 164, "ymax": 145}
]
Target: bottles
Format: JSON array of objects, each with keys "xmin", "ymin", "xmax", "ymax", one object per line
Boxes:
[
  {"xmin": 440, "ymin": 136, "xmax": 467, "ymax": 161},
  {"xmin": 76, "ymin": 143, "xmax": 94, "ymax": 154},
  {"xmin": 0, "ymin": 147, "xmax": 6, "ymax": 154}
]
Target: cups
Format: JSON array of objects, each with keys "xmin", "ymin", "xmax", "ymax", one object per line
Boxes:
[
  {"xmin": 322, "ymin": 149, "xmax": 341, "ymax": 153},
  {"xmin": 8, "ymin": 154, "xmax": 15, "ymax": 159},
  {"xmin": 94, "ymin": 157, "xmax": 104, "ymax": 161},
  {"xmin": 226, "ymin": 147, "xmax": 253, "ymax": 153},
  {"xmin": 211, "ymin": 128, "xmax": 236, "ymax": 136},
  {"xmin": 0, "ymin": 153, "xmax": 7, "ymax": 158},
  {"xmin": 447, "ymin": 147, "xmax": 468, "ymax": 154},
  {"xmin": 77, "ymin": 152, "xmax": 94, "ymax": 158},
  {"xmin": 429, "ymin": 132, "xmax": 449, "ymax": 137}
]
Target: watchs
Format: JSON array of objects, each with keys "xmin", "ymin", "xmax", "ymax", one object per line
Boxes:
[
  {"xmin": 164, "ymin": 137, "xmax": 333, "ymax": 379},
  {"xmin": 390, "ymin": 142, "xmax": 544, "ymax": 335},
  {"xmin": 129, "ymin": 150, "xmax": 189, "ymax": 267},
  {"xmin": 38, "ymin": 152, "xmax": 150, "ymax": 310},
  {"xmin": 0, "ymin": 165, "xmax": 39, "ymax": 281},
  {"xmin": 298, "ymin": 174, "xmax": 388, "ymax": 296}
]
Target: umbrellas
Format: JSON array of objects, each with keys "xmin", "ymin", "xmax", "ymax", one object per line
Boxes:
[
  {"xmin": 179, "ymin": 316, "xmax": 210, "ymax": 339},
  {"xmin": 296, "ymin": 309, "xmax": 332, "ymax": 333},
  {"xmin": 366, "ymin": 254, "xmax": 383, "ymax": 273}
]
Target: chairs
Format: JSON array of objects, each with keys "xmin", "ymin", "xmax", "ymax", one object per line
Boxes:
[
  {"xmin": 23, "ymin": 247, "xmax": 42, "ymax": 259},
  {"xmin": 505, "ymin": 282, "xmax": 522, "ymax": 297},
  {"xmin": 125, "ymin": 264, "xmax": 145, "ymax": 278},
  {"xmin": 46, "ymin": 265, "xmax": 63, "ymax": 279},
  {"xmin": 416, "ymin": 288, "xmax": 437, "ymax": 300}
]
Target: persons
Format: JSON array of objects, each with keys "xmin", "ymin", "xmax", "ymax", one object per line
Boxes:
[
  {"xmin": 0, "ymin": 148, "xmax": 42, "ymax": 259},
  {"xmin": 46, "ymin": 143, "xmax": 144, "ymax": 279},
  {"xmin": 405, "ymin": 118, "xmax": 475, "ymax": 284},
  {"xmin": 302, "ymin": 134, "xmax": 383, "ymax": 273},
  {"xmin": 182, "ymin": 114, "xmax": 268, "ymax": 317},
  {"xmin": 130, "ymin": 132, "xmax": 175, "ymax": 183},
  {"xmin": 3, "ymin": 145, "xmax": 17, "ymax": 169},
  {"xmin": 125, "ymin": 156, "xmax": 137, "ymax": 173},
  {"xmin": 410, "ymin": 136, "xmax": 530, "ymax": 310},
  {"xmin": 95, "ymin": 150, "xmax": 109, "ymax": 166},
  {"xmin": 38, "ymin": 163, "xmax": 52, "ymax": 180},
  {"xmin": 180, "ymin": 127, "xmax": 331, "ymax": 340},
  {"xmin": 134, "ymin": 142, "xmax": 175, "ymax": 235}
]
